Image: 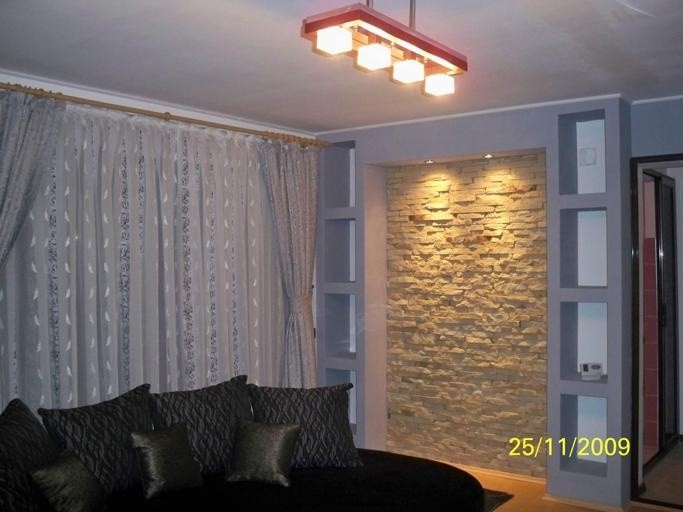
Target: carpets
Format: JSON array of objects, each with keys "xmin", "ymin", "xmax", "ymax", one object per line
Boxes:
[{"xmin": 483, "ymin": 488, "xmax": 514, "ymax": 512}]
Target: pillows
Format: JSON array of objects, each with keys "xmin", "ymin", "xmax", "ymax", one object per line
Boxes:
[
  {"xmin": 31, "ymin": 451, "xmax": 110, "ymax": 512},
  {"xmin": 243, "ymin": 381, "xmax": 363, "ymax": 473},
  {"xmin": 129, "ymin": 420, "xmax": 203, "ymax": 501},
  {"xmin": 38, "ymin": 382, "xmax": 157, "ymax": 499},
  {"xmin": 219, "ymin": 417, "xmax": 302, "ymax": 489},
  {"xmin": 145, "ymin": 375, "xmax": 252, "ymax": 487}
]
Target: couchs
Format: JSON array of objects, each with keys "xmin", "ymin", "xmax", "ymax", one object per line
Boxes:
[{"xmin": 0, "ymin": 397, "xmax": 484, "ymax": 512}]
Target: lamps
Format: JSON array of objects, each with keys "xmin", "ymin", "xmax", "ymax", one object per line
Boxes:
[{"xmin": 305, "ymin": 0, "xmax": 467, "ymax": 96}]
